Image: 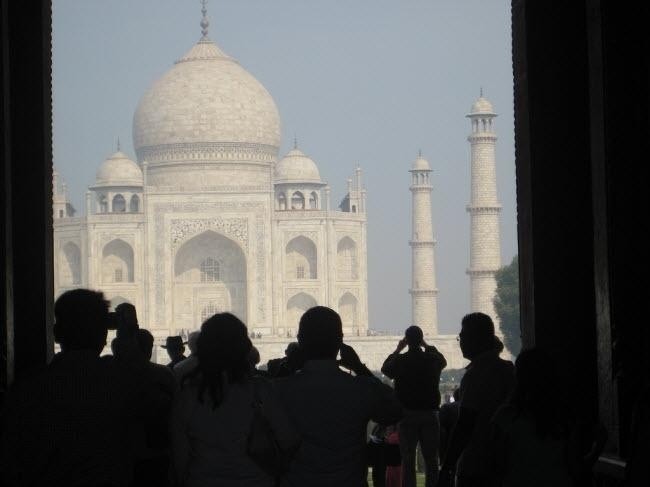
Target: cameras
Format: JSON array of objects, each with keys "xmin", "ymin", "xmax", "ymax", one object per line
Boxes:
[{"xmin": 108, "ymin": 312, "xmax": 121, "ymax": 331}]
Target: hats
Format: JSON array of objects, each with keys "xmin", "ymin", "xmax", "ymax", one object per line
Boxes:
[{"xmin": 159, "ymin": 334, "xmax": 189, "ymax": 349}]
